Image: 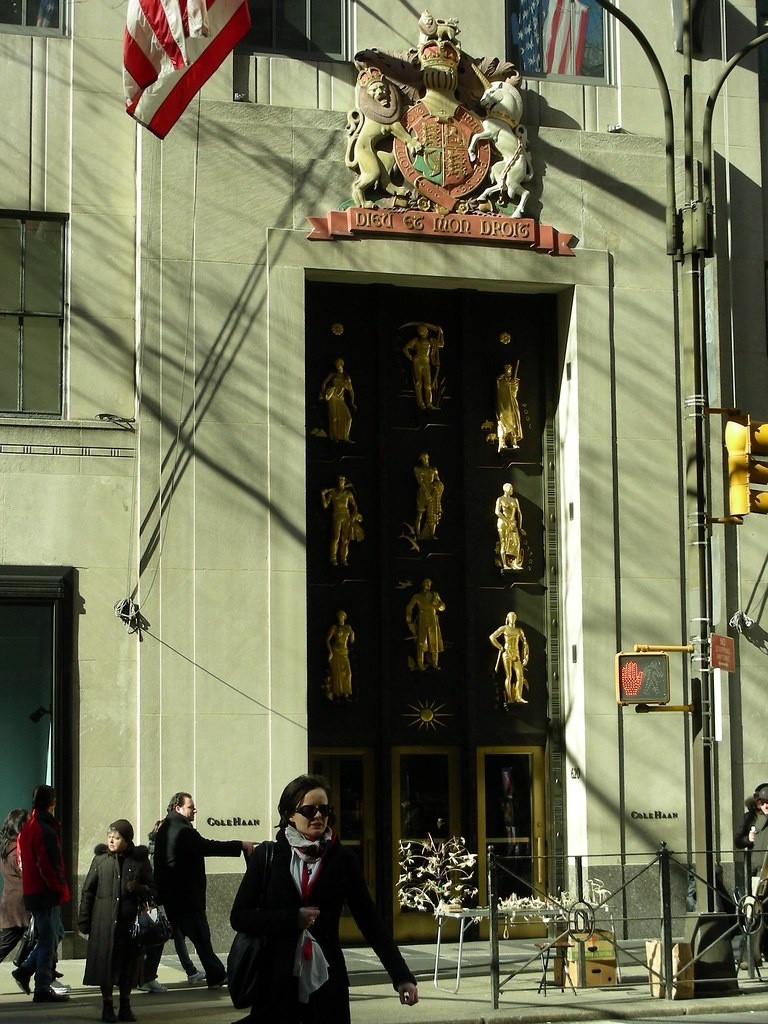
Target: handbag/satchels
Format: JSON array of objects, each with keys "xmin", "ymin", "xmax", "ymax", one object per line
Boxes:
[
  {"xmin": 13, "ymin": 914, "xmax": 37, "ymax": 968},
  {"xmin": 131, "ymin": 885, "xmax": 173, "ymax": 948},
  {"xmin": 226, "ymin": 839, "xmax": 274, "ymax": 1010}
]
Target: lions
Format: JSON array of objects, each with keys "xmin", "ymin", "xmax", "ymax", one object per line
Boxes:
[
  {"xmin": 344, "ymin": 72, "xmax": 423, "ymax": 208},
  {"xmin": 412, "ymin": 13, "xmax": 462, "ymax": 50}
]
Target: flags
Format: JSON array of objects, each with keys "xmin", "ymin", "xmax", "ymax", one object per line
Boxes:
[
  {"xmin": 123, "ymin": 0, "xmax": 251, "ymax": 140},
  {"xmin": 510, "ymin": 0, "xmax": 589, "ymax": 76}
]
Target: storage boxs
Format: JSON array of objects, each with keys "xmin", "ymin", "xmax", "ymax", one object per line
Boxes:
[{"xmin": 557, "ymin": 930, "xmax": 618, "ymax": 987}]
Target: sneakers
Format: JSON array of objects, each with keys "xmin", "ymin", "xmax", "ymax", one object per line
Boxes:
[
  {"xmin": 188, "ymin": 971, "xmax": 207, "ymax": 983},
  {"xmin": 52, "ymin": 981, "xmax": 71, "ymax": 994},
  {"xmin": 137, "ymin": 980, "xmax": 168, "ymax": 993},
  {"xmin": 207, "ymin": 974, "xmax": 229, "ymax": 990}
]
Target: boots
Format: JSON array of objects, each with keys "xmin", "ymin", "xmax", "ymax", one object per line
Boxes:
[
  {"xmin": 101, "ymin": 999, "xmax": 118, "ymax": 1024},
  {"xmin": 118, "ymin": 998, "xmax": 138, "ymax": 1022}
]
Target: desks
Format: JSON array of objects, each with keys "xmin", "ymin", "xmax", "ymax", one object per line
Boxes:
[{"xmin": 432, "ymin": 905, "xmax": 621, "ymax": 995}]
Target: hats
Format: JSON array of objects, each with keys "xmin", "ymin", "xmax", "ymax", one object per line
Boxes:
[
  {"xmin": 111, "ymin": 819, "xmax": 134, "ymax": 844},
  {"xmin": 759, "ymin": 787, "xmax": 768, "ymax": 800}
]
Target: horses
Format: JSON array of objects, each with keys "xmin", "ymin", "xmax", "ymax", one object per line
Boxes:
[{"xmin": 468, "ymin": 80, "xmax": 534, "ymax": 219}]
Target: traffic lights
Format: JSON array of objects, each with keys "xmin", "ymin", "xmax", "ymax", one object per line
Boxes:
[
  {"xmin": 722, "ymin": 415, "xmax": 768, "ymax": 517},
  {"xmin": 614, "ymin": 651, "xmax": 672, "ymax": 704}
]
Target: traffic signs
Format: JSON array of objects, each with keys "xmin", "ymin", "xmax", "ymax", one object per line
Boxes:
[{"xmin": 709, "ymin": 633, "xmax": 735, "ymax": 673}]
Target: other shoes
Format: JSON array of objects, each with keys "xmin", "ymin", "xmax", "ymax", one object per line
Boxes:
[
  {"xmin": 11, "ymin": 967, "xmax": 32, "ymax": 996},
  {"xmin": 32, "ymin": 983, "xmax": 70, "ymax": 1002},
  {"xmin": 740, "ymin": 960, "xmax": 762, "ymax": 969}
]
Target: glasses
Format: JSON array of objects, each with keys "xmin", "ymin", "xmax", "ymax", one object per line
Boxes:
[
  {"xmin": 760, "ymin": 800, "xmax": 768, "ymax": 805},
  {"xmin": 753, "ymin": 794, "xmax": 759, "ymax": 799},
  {"xmin": 294, "ymin": 804, "xmax": 334, "ymax": 818}
]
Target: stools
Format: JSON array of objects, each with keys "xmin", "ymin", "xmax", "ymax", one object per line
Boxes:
[{"xmin": 533, "ymin": 942, "xmax": 576, "ymax": 996}]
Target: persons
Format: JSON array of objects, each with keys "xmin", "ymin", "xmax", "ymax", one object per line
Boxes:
[
  {"xmin": 326, "ymin": 610, "xmax": 355, "ymax": 700},
  {"xmin": 414, "ymin": 453, "xmax": 444, "ymax": 538},
  {"xmin": 227, "ymin": 774, "xmax": 419, "ymax": 1024},
  {"xmin": 495, "ymin": 482, "xmax": 527, "ymax": 569},
  {"xmin": 320, "ymin": 359, "xmax": 356, "ymax": 441},
  {"xmin": 0, "ymin": 785, "xmax": 72, "ymax": 1003},
  {"xmin": 489, "ymin": 612, "xmax": 529, "ymax": 703},
  {"xmin": 405, "ymin": 578, "xmax": 445, "ymax": 669},
  {"xmin": 497, "ymin": 364, "xmax": 522, "ymax": 451},
  {"xmin": 138, "ymin": 791, "xmax": 261, "ymax": 993},
  {"xmin": 322, "ymin": 476, "xmax": 362, "ymax": 566},
  {"xmin": 400, "ymin": 322, "xmax": 444, "ymax": 409},
  {"xmin": 734, "ymin": 782, "xmax": 768, "ymax": 969},
  {"xmin": 77, "ymin": 818, "xmax": 155, "ymax": 1024}
]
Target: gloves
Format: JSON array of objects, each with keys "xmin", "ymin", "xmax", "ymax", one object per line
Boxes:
[
  {"xmin": 127, "ymin": 881, "xmax": 139, "ymax": 893},
  {"xmin": 79, "ymin": 923, "xmax": 91, "ymax": 934}
]
